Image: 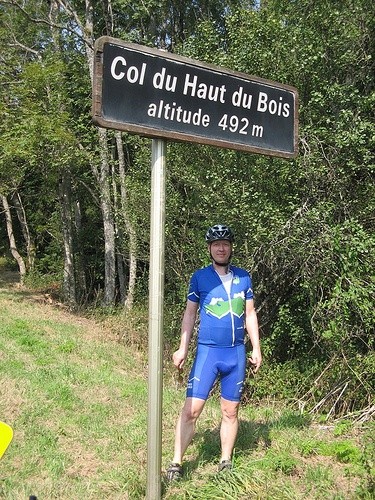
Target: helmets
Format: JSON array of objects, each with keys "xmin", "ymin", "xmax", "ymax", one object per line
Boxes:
[{"xmin": 205, "ymin": 224, "xmax": 235, "ymax": 244}]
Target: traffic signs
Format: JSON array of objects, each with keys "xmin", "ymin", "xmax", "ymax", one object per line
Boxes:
[{"xmin": 87, "ymin": 36, "xmax": 300, "ymax": 161}]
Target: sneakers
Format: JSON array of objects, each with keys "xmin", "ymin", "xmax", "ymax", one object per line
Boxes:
[
  {"xmin": 218, "ymin": 460, "xmax": 231, "ymax": 472},
  {"xmin": 166, "ymin": 462, "xmax": 183, "ymax": 483}
]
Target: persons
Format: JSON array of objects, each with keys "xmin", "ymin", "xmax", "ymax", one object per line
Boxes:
[{"xmin": 166, "ymin": 224, "xmax": 261, "ymax": 478}]
